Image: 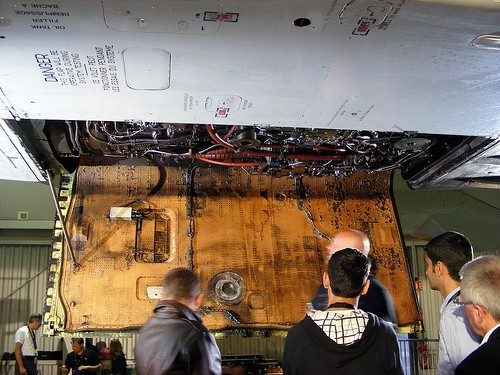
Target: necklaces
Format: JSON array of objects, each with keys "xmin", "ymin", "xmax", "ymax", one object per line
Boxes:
[{"xmin": 329, "ymin": 302, "xmax": 355, "ymax": 310}]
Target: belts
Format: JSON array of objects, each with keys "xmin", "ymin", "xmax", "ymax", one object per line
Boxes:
[{"xmin": 22, "ymin": 356, "xmax": 35, "ymax": 359}]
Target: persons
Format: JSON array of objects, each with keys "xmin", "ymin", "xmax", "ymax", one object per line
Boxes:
[
  {"xmin": 454, "ymin": 254, "xmax": 500, "ymax": 375},
  {"xmin": 85, "ymin": 338, "xmax": 128, "ymax": 375},
  {"xmin": 59, "ymin": 338, "xmax": 102, "ymax": 375},
  {"xmin": 14, "ymin": 313, "xmax": 42, "ymax": 375},
  {"xmin": 309, "ymin": 229, "xmax": 398, "ymax": 329},
  {"xmin": 282, "ymin": 248, "xmax": 404, "ymax": 375},
  {"xmin": 134, "ymin": 268, "xmax": 222, "ymax": 375},
  {"xmin": 423, "ymin": 231, "xmax": 483, "ymax": 375}
]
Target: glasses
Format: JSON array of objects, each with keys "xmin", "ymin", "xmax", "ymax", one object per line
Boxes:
[{"xmin": 453, "ymin": 297, "xmax": 487, "ymax": 315}]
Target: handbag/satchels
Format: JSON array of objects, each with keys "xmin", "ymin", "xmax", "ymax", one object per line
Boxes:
[{"xmin": 2, "ymin": 352, "xmax": 16, "ymax": 360}]
